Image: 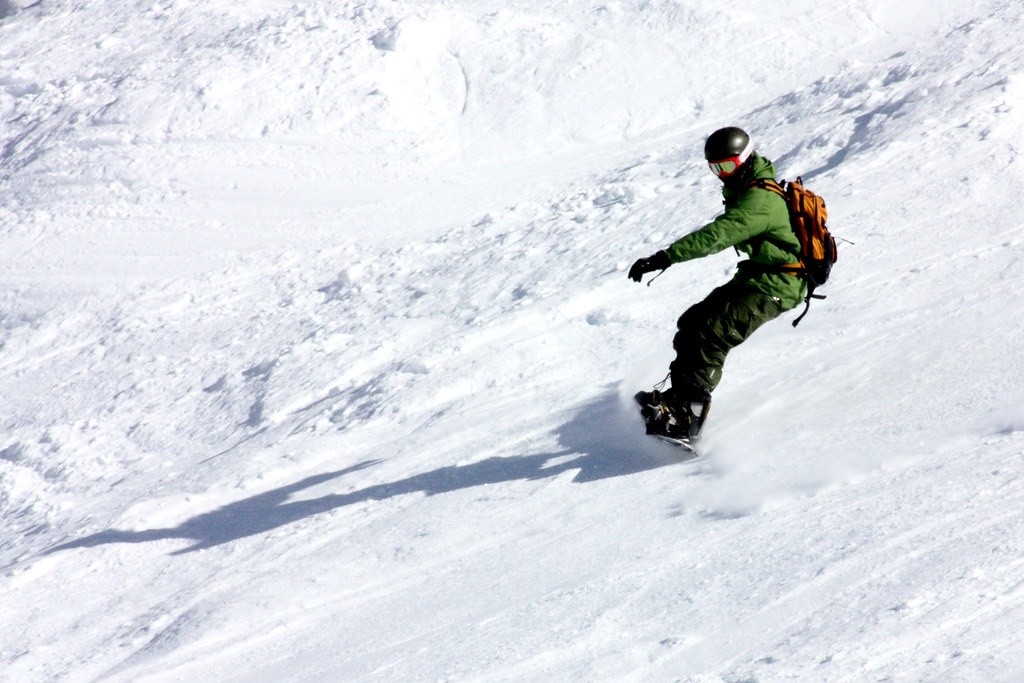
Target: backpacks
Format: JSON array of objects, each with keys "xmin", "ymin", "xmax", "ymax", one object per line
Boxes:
[{"xmin": 720, "ymin": 176, "xmax": 838, "ymax": 327}]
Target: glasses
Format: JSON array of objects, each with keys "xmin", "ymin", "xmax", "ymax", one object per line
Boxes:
[{"xmin": 708, "ymin": 140, "xmax": 753, "ymax": 177}]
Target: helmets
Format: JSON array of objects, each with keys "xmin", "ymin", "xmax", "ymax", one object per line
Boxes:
[{"xmin": 704, "ymin": 126, "xmax": 755, "ymax": 188}]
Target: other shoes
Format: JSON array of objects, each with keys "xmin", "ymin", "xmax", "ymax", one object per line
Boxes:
[
  {"xmin": 643, "ymin": 402, "xmax": 701, "ymax": 440},
  {"xmin": 635, "ymin": 386, "xmax": 674, "ymax": 410}
]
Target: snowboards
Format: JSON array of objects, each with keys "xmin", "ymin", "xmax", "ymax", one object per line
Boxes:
[{"xmin": 635, "ymin": 390, "xmax": 700, "ymax": 459}]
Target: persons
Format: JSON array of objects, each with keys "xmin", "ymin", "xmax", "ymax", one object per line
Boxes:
[{"xmin": 628, "ymin": 127, "xmax": 806, "ymax": 444}]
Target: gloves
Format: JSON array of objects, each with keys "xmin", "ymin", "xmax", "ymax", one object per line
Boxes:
[{"xmin": 628, "ymin": 249, "xmax": 673, "ymax": 286}]
